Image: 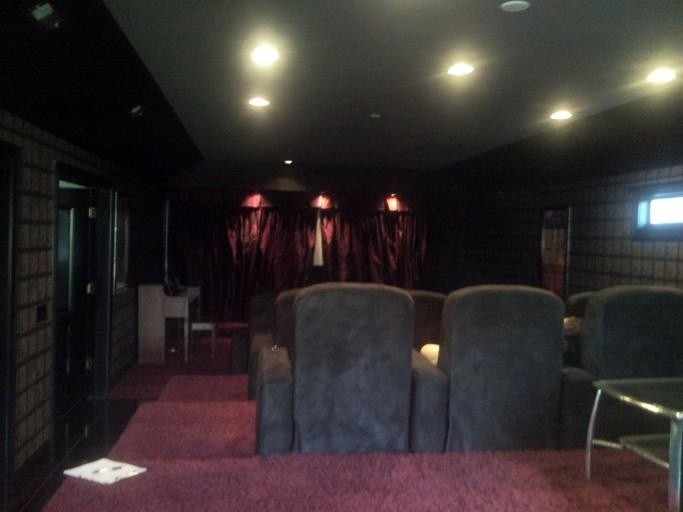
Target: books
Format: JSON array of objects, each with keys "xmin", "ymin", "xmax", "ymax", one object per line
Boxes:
[{"xmin": 63, "ymin": 457, "xmax": 147, "ymax": 485}]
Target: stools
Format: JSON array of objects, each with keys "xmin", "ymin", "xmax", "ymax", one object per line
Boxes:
[{"xmin": 191, "ymin": 312, "xmax": 218, "ymax": 359}]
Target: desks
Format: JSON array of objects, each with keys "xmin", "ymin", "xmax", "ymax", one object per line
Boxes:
[{"xmin": 584, "ymin": 377, "xmax": 682, "ymax": 512}]
